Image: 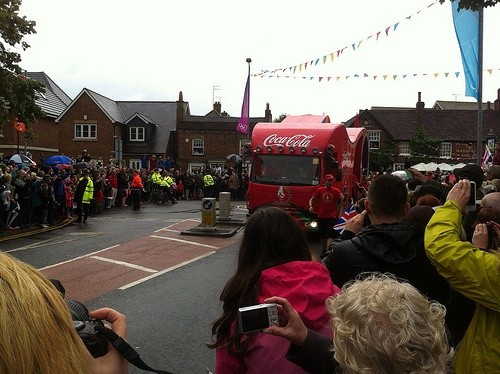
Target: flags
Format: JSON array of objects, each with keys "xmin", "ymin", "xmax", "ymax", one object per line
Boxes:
[
  {"xmin": 482, "ymin": 149, "xmax": 492, "ymax": 169},
  {"xmin": 14, "ymin": 67, "xmax": 27, "ymax": 133},
  {"xmin": 236, "ymin": 76, "xmax": 251, "ymax": 135},
  {"xmin": 451, "ymin": 0, "xmax": 478, "ymax": 101},
  {"xmin": 334, "ymin": 198, "xmax": 357, "ymax": 234}
]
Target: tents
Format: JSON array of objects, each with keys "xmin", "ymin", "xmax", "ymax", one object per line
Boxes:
[{"xmin": 411, "ymin": 161, "xmax": 472, "ymax": 172}]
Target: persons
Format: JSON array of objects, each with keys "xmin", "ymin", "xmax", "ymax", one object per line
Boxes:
[
  {"xmin": 0, "ymin": 252, "xmax": 130, "ymax": 374},
  {"xmin": 0, "ymin": 153, "xmax": 500, "ymax": 352},
  {"xmin": 207, "ymin": 207, "xmax": 342, "ymax": 373},
  {"xmin": 263, "ymin": 273, "xmax": 449, "ymax": 373},
  {"xmin": 424, "ymin": 179, "xmax": 500, "ymax": 374}
]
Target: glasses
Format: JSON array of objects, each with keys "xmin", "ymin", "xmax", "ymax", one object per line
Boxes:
[{"xmin": 458, "ymin": 176, "xmax": 468, "ymax": 180}]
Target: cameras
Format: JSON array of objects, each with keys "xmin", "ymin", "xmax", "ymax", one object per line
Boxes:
[
  {"xmin": 48, "ymin": 279, "xmax": 110, "ymax": 358},
  {"xmin": 363, "ymin": 213, "xmax": 371, "ymax": 228},
  {"xmin": 237, "ymin": 303, "xmax": 280, "ymax": 335}
]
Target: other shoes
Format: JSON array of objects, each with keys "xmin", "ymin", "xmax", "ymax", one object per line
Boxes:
[
  {"xmin": 172, "ymin": 202, "xmax": 178, "ymax": 204},
  {"xmin": 105, "ymin": 206, "xmax": 111, "ymax": 208},
  {"xmin": 122, "ymin": 204, "xmax": 128, "ymax": 206}
]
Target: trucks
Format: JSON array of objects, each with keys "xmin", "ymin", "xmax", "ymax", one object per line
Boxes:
[{"xmin": 244, "ymin": 114, "xmax": 371, "ymax": 238}]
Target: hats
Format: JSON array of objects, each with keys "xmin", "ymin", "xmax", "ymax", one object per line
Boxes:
[
  {"xmin": 453, "ymin": 164, "xmax": 484, "ymax": 190},
  {"xmin": 485, "ymin": 165, "xmax": 500, "ymax": 175},
  {"xmin": 325, "ymin": 174, "xmax": 334, "ymax": 180}
]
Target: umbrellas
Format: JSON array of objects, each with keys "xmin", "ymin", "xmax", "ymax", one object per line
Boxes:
[
  {"xmin": 225, "ymin": 154, "xmax": 243, "ymax": 161},
  {"xmin": 46, "ymin": 157, "xmax": 72, "ymax": 165},
  {"xmin": 3, "ymin": 154, "xmax": 34, "ymax": 165}
]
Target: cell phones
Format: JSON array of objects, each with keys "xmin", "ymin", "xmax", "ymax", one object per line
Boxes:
[{"xmin": 464, "ymin": 181, "xmax": 477, "ymax": 212}]
